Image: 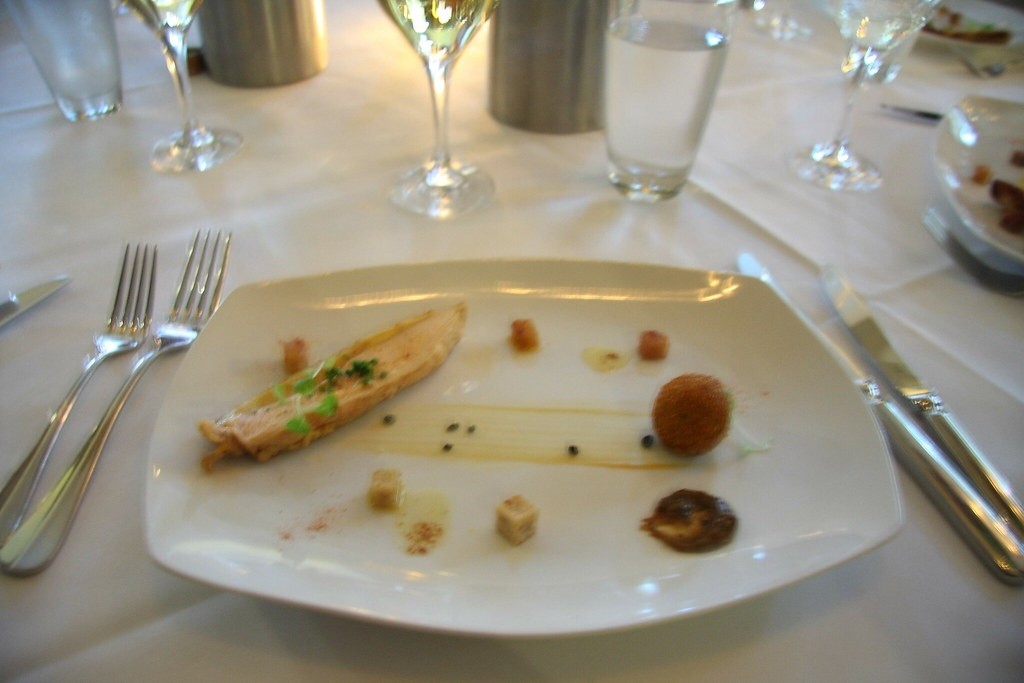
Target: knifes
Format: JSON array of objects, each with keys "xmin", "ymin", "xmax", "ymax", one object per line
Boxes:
[
  {"xmin": 0, "ymin": 276, "xmax": 72, "ymax": 328},
  {"xmin": 818, "ymin": 269, "xmax": 1024, "ymax": 541},
  {"xmin": 736, "ymin": 249, "xmax": 1023, "ymax": 587}
]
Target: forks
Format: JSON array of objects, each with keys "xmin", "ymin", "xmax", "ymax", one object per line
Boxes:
[
  {"xmin": 0, "ymin": 229, "xmax": 235, "ymax": 577},
  {"xmin": 960, "ymin": 57, "xmax": 1024, "ymax": 79},
  {"xmin": 0, "ymin": 243, "xmax": 159, "ymax": 554}
]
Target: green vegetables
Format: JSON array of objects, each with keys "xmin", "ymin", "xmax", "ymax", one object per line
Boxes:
[{"xmin": 270, "ymin": 353, "xmax": 387, "ymax": 437}]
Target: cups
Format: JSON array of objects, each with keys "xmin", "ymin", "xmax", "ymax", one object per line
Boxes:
[
  {"xmin": 484, "ymin": 0, "xmax": 641, "ymax": 135},
  {"xmin": 1, "ymin": 0, "xmax": 123, "ymax": 122},
  {"xmin": 602, "ymin": 1, "xmax": 736, "ymax": 198},
  {"xmin": 752, "ymin": 1, "xmax": 828, "ymax": 41},
  {"xmin": 195, "ymin": 0, "xmax": 332, "ymax": 90}
]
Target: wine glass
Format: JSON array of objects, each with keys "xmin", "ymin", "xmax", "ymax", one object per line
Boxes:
[
  {"xmin": 791, "ymin": 0, "xmax": 938, "ymax": 193},
  {"xmin": 121, "ymin": 0, "xmax": 244, "ymax": 173},
  {"xmin": 377, "ymin": 0, "xmax": 502, "ymax": 218}
]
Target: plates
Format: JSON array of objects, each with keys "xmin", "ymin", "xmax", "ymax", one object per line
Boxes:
[
  {"xmin": 139, "ymin": 261, "xmax": 905, "ymax": 641},
  {"xmin": 815, "ymin": 1, "xmax": 1024, "ymax": 56},
  {"xmin": 930, "ymin": 93, "xmax": 1023, "ymax": 275}
]
